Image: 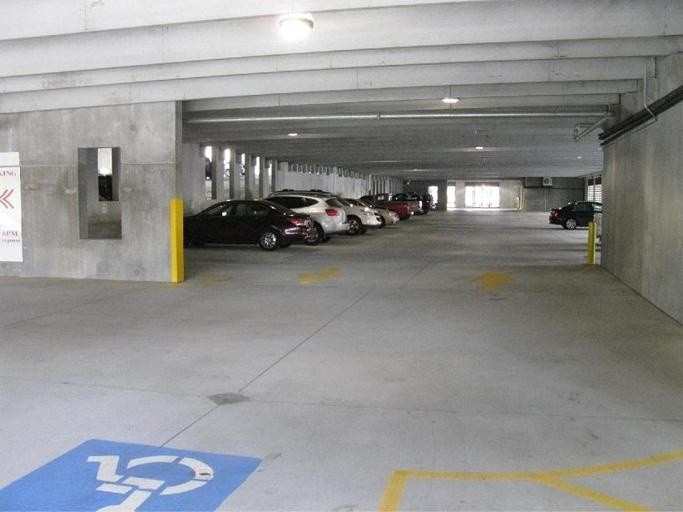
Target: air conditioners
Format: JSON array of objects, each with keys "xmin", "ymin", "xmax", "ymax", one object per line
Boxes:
[{"xmin": 542, "ymin": 176, "xmax": 553, "ymax": 187}]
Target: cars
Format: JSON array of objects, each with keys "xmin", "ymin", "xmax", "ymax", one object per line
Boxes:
[
  {"xmin": 549, "ymin": 199, "xmax": 601, "ymax": 232},
  {"xmin": 205, "ymin": 152, "xmax": 246, "ymax": 179},
  {"xmin": 182, "ymin": 189, "xmax": 436, "ymax": 250}
]
